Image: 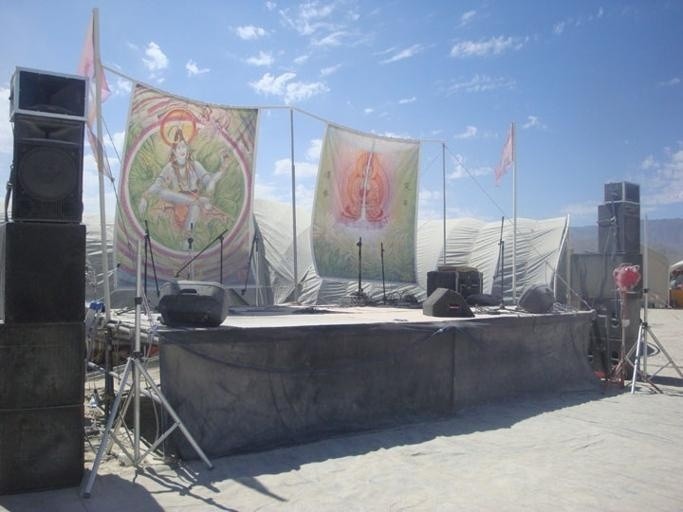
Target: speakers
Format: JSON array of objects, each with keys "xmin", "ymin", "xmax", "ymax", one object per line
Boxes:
[
  {"xmin": 158, "ymin": 281, "xmax": 228, "ymax": 327},
  {"xmin": 423, "ymin": 287, "xmax": 475, "ymax": 317},
  {"xmin": 519, "ymin": 283, "xmax": 556, "ymax": 313},
  {"xmin": 570, "ymin": 202, "xmax": 643, "ymax": 380},
  {"xmin": 0, "ymin": 113, "xmax": 87, "ymax": 498}
]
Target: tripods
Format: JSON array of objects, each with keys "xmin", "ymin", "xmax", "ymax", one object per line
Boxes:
[
  {"xmin": 83, "ymin": 239, "xmax": 214, "ymax": 500},
  {"xmin": 116, "ymin": 221, "xmax": 160, "ymax": 315},
  {"xmin": 493, "ymin": 217, "xmax": 515, "ymax": 311},
  {"xmin": 603, "ymin": 215, "xmax": 683, "ymax": 395}
]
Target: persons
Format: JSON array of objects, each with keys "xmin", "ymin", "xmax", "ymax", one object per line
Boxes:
[{"xmin": 138, "ymin": 129, "xmax": 232, "ymax": 251}]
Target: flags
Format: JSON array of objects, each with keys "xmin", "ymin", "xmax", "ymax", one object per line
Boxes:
[{"xmin": 495, "ymin": 132, "xmax": 513, "ymax": 186}]
